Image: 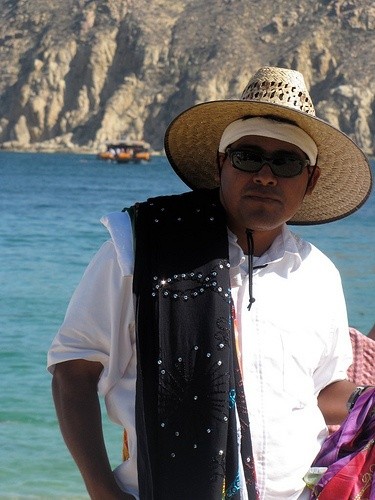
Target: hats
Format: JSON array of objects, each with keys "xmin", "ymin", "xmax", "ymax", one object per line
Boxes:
[{"xmin": 163, "ymin": 65, "xmax": 374, "ymax": 227}]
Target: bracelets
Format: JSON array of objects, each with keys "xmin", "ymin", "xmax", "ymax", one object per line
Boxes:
[{"xmin": 346, "ymin": 387, "xmax": 366, "ymax": 412}]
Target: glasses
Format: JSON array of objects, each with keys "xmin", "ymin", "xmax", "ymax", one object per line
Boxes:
[{"xmin": 224, "ymin": 147, "xmax": 310, "ymax": 178}]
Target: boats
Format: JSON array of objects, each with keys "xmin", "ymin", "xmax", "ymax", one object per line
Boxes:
[{"xmin": 96, "ymin": 139, "xmax": 151, "ymax": 164}]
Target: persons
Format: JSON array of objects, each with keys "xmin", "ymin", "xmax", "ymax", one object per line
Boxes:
[{"xmin": 48, "ymin": 66, "xmax": 375, "ymax": 500}]
